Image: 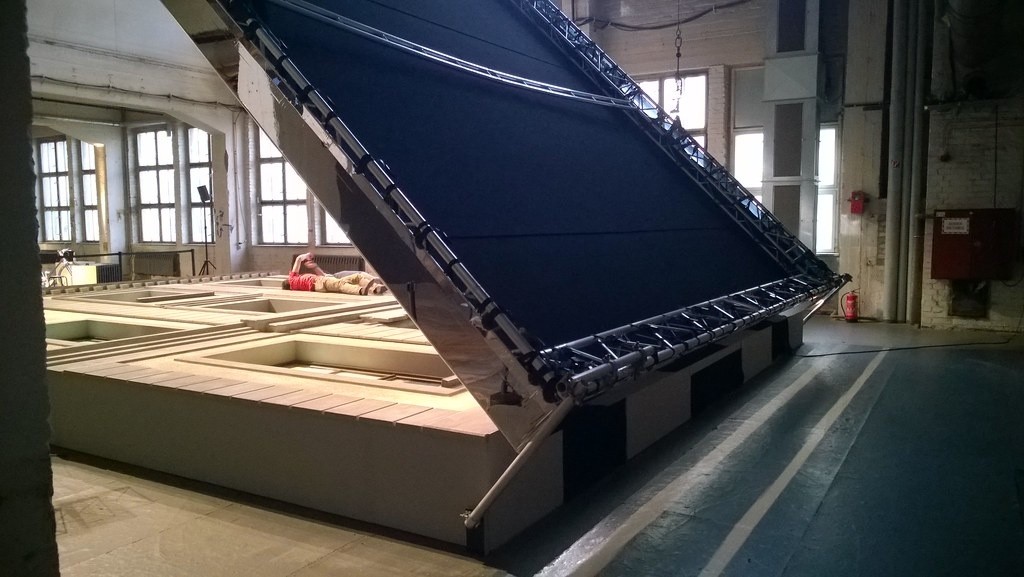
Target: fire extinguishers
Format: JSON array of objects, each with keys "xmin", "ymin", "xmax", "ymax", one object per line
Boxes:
[{"xmin": 840, "ymin": 288, "xmax": 861, "ymax": 323}]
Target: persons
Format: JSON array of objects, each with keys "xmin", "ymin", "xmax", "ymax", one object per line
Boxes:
[{"xmin": 279, "ymin": 254, "xmax": 385, "ymax": 296}]
